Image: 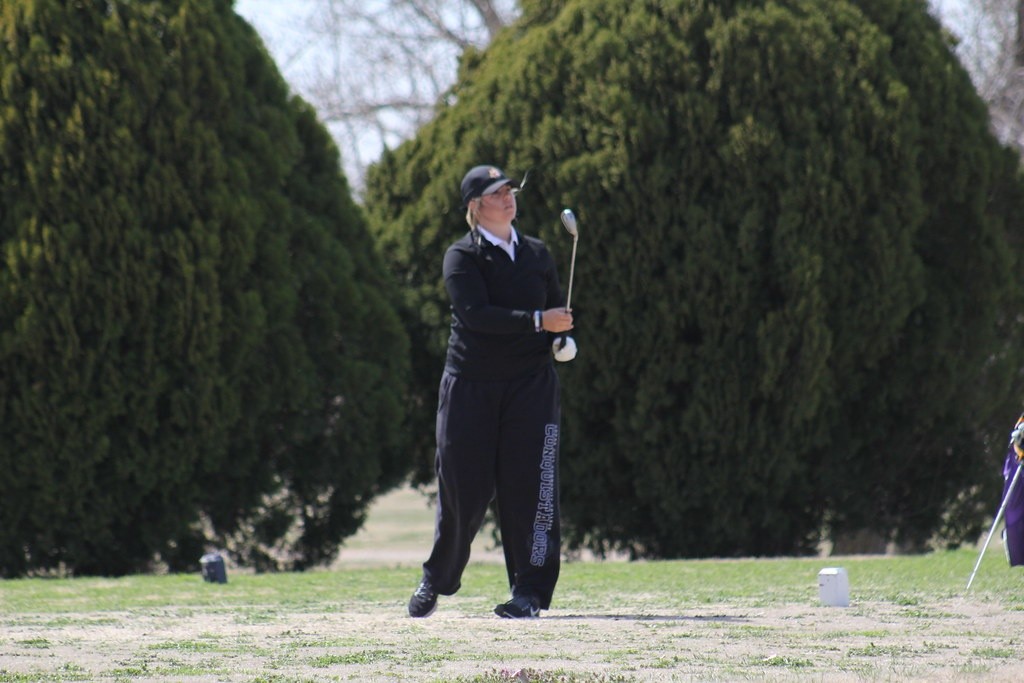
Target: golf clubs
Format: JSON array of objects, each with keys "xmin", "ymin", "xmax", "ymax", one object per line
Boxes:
[{"xmin": 560, "ymin": 208, "xmax": 579, "ymax": 351}]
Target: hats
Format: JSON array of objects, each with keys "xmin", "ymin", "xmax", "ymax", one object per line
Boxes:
[{"xmin": 462, "ymin": 165, "xmax": 513, "ymax": 204}]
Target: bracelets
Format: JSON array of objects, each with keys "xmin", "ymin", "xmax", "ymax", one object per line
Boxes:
[{"xmin": 534, "ymin": 310, "xmax": 540, "ymax": 333}]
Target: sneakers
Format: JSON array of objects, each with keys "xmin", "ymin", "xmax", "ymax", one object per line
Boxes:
[
  {"xmin": 494, "ymin": 597, "xmax": 540, "ymax": 619},
  {"xmin": 409, "ymin": 577, "xmax": 438, "ymax": 618}
]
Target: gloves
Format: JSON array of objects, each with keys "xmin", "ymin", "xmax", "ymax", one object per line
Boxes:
[{"xmin": 552, "ymin": 337, "xmax": 578, "ymax": 362}]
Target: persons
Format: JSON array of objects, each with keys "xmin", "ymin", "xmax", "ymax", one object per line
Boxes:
[{"xmin": 412, "ymin": 167, "xmax": 583, "ymax": 620}]
